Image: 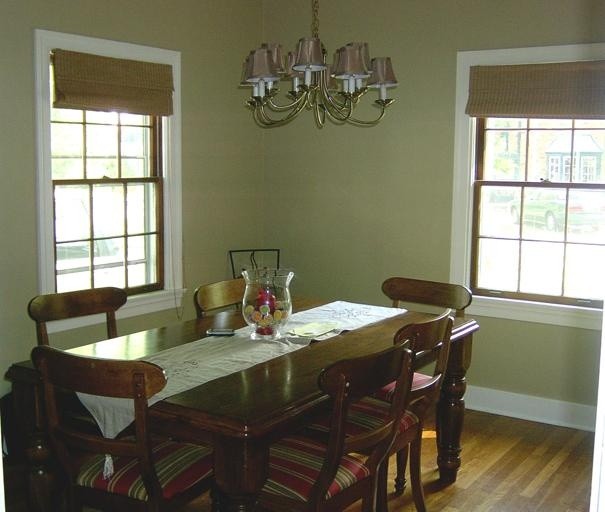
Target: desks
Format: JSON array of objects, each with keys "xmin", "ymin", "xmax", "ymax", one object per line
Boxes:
[{"xmin": 4, "ymin": 297, "xmax": 478, "ymax": 511}]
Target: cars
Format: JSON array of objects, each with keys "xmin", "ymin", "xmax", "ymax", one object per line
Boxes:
[{"xmin": 509, "ymin": 193, "xmax": 590, "ymax": 233}]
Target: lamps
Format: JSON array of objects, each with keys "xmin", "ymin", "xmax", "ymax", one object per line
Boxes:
[{"xmin": 238, "ymin": 1, "xmax": 398, "ymax": 131}]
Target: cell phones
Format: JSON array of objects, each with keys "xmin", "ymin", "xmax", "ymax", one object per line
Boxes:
[{"xmin": 206, "ymin": 328, "xmax": 234, "ymax": 335}]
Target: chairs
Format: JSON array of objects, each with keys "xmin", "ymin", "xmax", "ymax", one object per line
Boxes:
[
  {"xmin": 216, "ymin": 339, "xmax": 415, "ymax": 510},
  {"xmin": 28, "ymin": 286, "xmax": 155, "ymax": 436},
  {"xmin": 320, "ymin": 278, "xmax": 475, "ymax": 494},
  {"xmin": 27, "ymin": 343, "xmax": 215, "ymax": 508},
  {"xmin": 299, "ymin": 307, "xmax": 457, "ymax": 511},
  {"xmin": 193, "ymin": 277, "xmax": 270, "ymax": 317}
]
